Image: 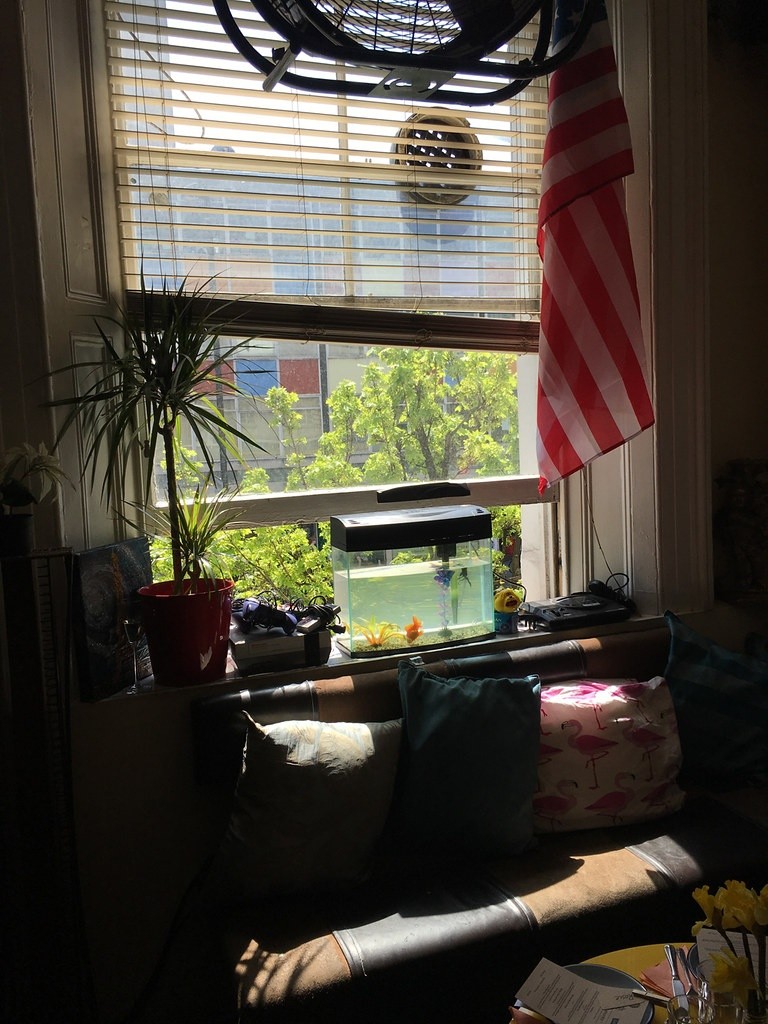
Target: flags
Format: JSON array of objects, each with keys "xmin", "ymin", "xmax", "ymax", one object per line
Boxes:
[{"xmin": 536, "ymin": 1, "xmax": 655, "ymax": 494}]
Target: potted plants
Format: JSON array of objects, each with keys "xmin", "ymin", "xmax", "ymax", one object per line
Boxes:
[{"xmin": 22, "ymin": 256, "xmax": 273, "ymax": 689}]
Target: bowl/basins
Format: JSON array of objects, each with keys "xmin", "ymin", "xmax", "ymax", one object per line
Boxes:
[{"xmin": 687, "ymin": 942, "xmax": 737, "ymax": 1007}]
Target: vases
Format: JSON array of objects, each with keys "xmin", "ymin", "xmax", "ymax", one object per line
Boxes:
[
  {"xmin": 742, "ymin": 980, "xmax": 768, "ymax": 1024},
  {"xmin": 0, "ymin": 514, "xmax": 34, "ymax": 557}
]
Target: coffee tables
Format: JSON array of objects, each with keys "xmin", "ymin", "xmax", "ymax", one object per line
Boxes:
[{"xmin": 506, "ymin": 942, "xmax": 752, "ymax": 1024}]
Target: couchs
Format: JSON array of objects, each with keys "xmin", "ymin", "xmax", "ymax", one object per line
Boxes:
[{"xmin": 191, "ymin": 607, "xmax": 768, "ymax": 1023}]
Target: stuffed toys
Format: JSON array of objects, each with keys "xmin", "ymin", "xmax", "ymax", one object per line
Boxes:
[{"xmin": 494, "ymin": 589, "xmax": 523, "ymax": 613}]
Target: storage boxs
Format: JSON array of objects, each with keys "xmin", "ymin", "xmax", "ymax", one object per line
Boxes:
[{"xmin": 330, "ymin": 504, "xmax": 497, "ymax": 660}]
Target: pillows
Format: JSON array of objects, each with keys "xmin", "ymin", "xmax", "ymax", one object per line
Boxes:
[
  {"xmin": 660, "ymin": 610, "xmax": 767, "ymax": 790},
  {"xmin": 204, "ymin": 710, "xmax": 403, "ymax": 905},
  {"xmin": 536, "ymin": 677, "xmax": 685, "ymax": 832},
  {"xmin": 395, "ymin": 654, "xmax": 539, "ymax": 868}
]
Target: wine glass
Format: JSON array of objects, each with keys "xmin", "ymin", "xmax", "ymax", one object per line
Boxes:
[{"xmin": 118, "ymin": 598, "xmax": 152, "ymax": 695}]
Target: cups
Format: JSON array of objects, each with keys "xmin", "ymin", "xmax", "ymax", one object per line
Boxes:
[
  {"xmin": 495, "ymin": 611, "xmax": 519, "ymax": 633},
  {"xmin": 667, "ymin": 994, "xmax": 717, "ymax": 1024}
]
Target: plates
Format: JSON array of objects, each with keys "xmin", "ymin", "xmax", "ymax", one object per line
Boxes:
[{"xmin": 562, "ymin": 963, "xmax": 655, "ymax": 1024}]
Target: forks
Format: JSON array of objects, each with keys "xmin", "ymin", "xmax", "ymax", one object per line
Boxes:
[{"xmin": 676, "ymin": 948, "xmax": 701, "ymax": 1003}]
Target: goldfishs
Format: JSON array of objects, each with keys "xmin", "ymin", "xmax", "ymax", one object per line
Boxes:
[{"xmin": 404, "ymin": 615, "xmax": 423, "ymax": 644}]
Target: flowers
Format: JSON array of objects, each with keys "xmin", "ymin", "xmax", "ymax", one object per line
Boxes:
[
  {"xmin": 687, "ymin": 879, "xmax": 768, "ymax": 1018},
  {"xmin": 0, "ymin": 439, "xmax": 78, "ymax": 514}
]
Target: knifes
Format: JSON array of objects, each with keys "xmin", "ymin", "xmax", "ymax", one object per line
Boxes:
[{"xmin": 663, "ymin": 944, "xmax": 690, "ymax": 1012}]
